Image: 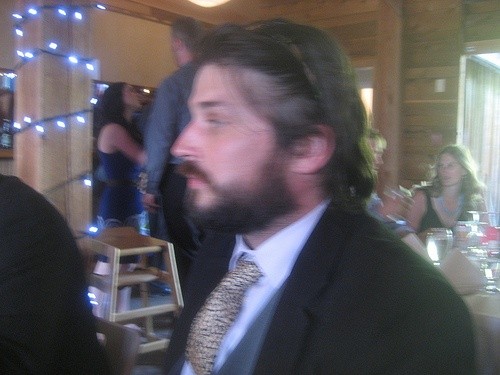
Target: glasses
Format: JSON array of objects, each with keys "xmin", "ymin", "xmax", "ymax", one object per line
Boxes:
[{"xmin": 243, "ymin": 21, "xmax": 334, "ymax": 121}]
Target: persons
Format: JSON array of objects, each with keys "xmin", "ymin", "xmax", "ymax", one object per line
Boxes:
[
  {"xmin": 92, "ymin": 17, "xmax": 207, "ymax": 341},
  {"xmin": 366, "ymin": 128, "xmax": 491, "ymax": 247},
  {"xmin": 164, "ymin": 16, "xmax": 475, "ymax": 375}
]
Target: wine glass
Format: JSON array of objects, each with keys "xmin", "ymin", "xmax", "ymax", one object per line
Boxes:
[
  {"xmin": 487, "ymin": 240, "xmax": 500, "ymax": 278},
  {"xmin": 465, "ymin": 210, "xmax": 489, "ymax": 234},
  {"xmin": 480, "ymin": 259, "xmax": 500, "ymax": 294},
  {"xmin": 465, "ymin": 222, "xmax": 488, "ymax": 257},
  {"xmin": 426, "ymin": 228, "xmax": 453, "ymax": 270}
]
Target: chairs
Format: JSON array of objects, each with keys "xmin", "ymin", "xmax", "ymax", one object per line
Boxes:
[{"xmin": 79, "ymin": 227, "xmax": 186, "ymax": 359}]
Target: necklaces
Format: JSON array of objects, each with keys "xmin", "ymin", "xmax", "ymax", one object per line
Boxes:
[{"xmin": 437, "ymin": 193, "xmax": 463, "ymax": 216}]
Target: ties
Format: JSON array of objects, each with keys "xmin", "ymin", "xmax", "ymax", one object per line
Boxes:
[{"xmin": 186, "ymin": 259, "xmax": 264, "ymax": 375}]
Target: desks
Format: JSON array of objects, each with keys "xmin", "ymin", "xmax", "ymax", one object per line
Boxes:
[{"xmin": 460, "ymin": 294, "xmax": 500, "ymax": 375}]
